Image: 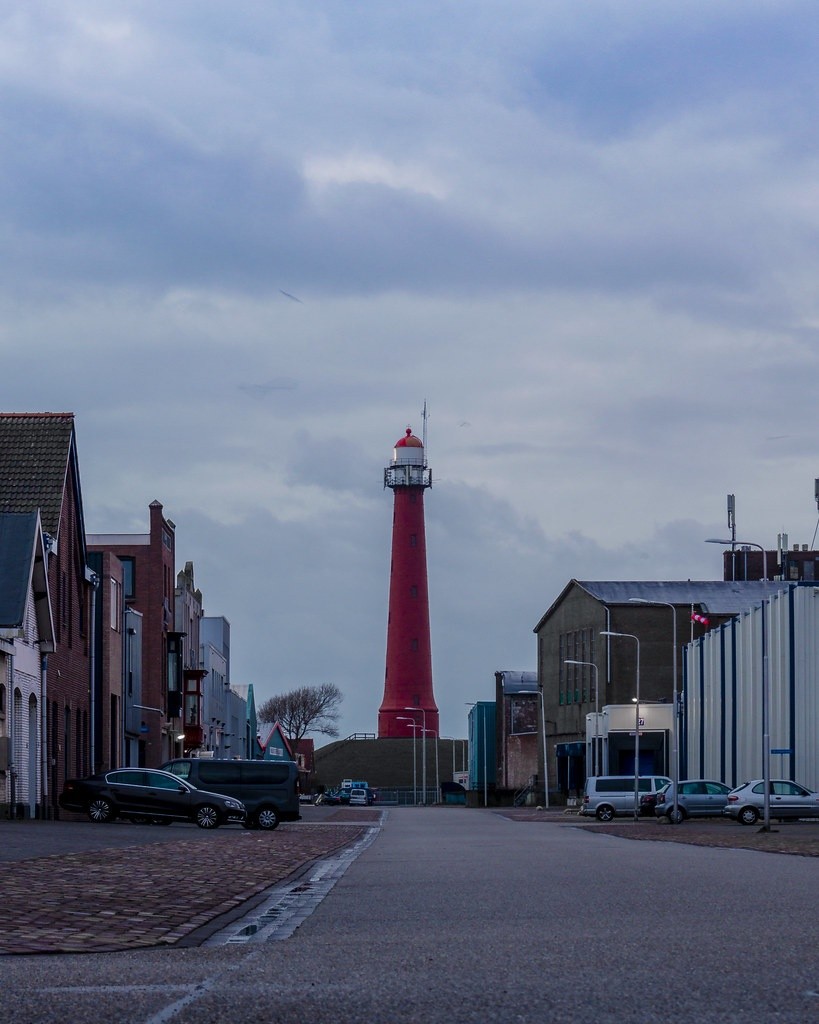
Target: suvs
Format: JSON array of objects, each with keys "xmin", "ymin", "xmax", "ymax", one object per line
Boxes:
[{"xmin": 654, "ymin": 779, "xmax": 734, "ymax": 824}]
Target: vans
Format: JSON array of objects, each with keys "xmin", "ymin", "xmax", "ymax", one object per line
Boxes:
[
  {"xmin": 582, "ymin": 775, "xmax": 674, "ymax": 821},
  {"xmin": 157, "ymin": 757, "xmax": 303, "ymax": 830},
  {"xmin": 333, "ymin": 788, "xmax": 374, "ymax": 807}
]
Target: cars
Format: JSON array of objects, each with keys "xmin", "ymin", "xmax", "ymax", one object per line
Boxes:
[
  {"xmin": 639, "ymin": 782, "xmax": 722, "ymax": 819},
  {"xmin": 300, "ymin": 792, "xmax": 350, "ymax": 806},
  {"xmin": 58, "ymin": 767, "xmax": 247, "ymax": 829},
  {"xmin": 724, "ymin": 779, "xmax": 819, "ymax": 825}
]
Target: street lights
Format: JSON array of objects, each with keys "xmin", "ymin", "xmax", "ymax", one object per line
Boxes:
[
  {"xmin": 704, "ymin": 537, "xmax": 770, "ymax": 833},
  {"xmin": 518, "ymin": 683, "xmax": 549, "ymax": 812},
  {"xmin": 629, "ymin": 597, "xmax": 678, "ymax": 826},
  {"xmin": 396, "ymin": 707, "xmax": 465, "ymax": 806},
  {"xmin": 464, "ymin": 702, "xmax": 488, "ymax": 807},
  {"xmin": 600, "ymin": 631, "xmax": 640, "ymax": 822},
  {"xmin": 564, "ymin": 660, "xmax": 599, "ymax": 777}
]
